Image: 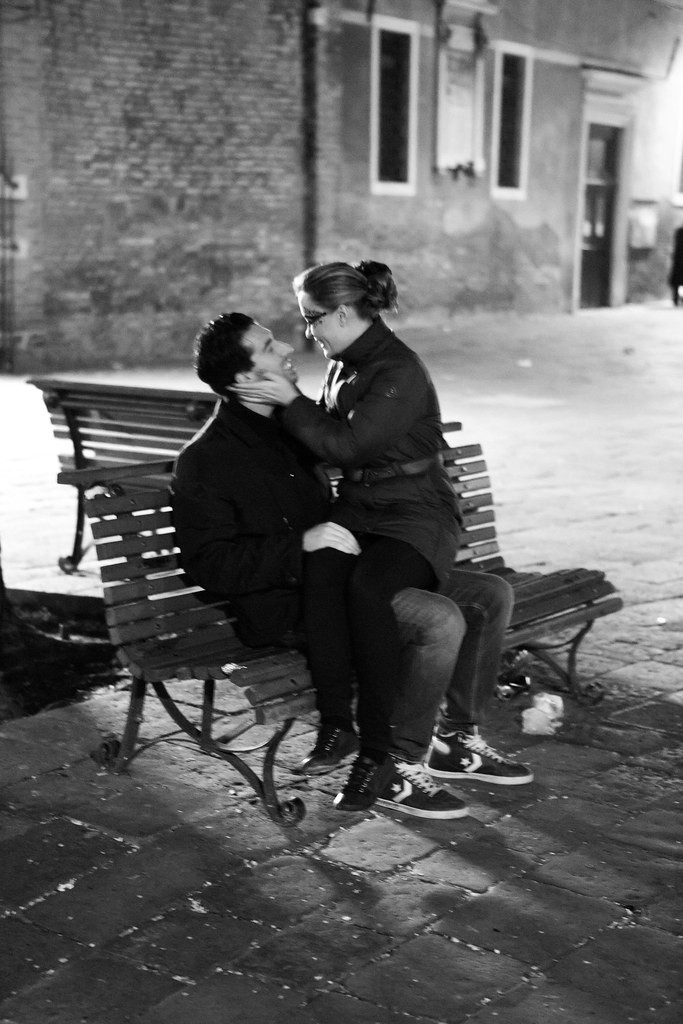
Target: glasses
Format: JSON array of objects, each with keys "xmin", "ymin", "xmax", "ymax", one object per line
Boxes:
[{"xmin": 304, "ymin": 311, "xmax": 328, "ymax": 327}]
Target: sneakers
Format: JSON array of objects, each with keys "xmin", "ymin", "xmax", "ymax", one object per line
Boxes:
[
  {"xmin": 374, "ymin": 757, "xmax": 470, "ymax": 820},
  {"xmin": 424, "ymin": 725, "xmax": 534, "ymax": 785},
  {"xmin": 295, "ymin": 726, "xmax": 361, "ymax": 776},
  {"xmin": 332, "ymin": 753, "xmax": 395, "ymax": 815}
]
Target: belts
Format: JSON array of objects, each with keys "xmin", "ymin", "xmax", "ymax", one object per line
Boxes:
[{"xmin": 340, "ymin": 453, "xmax": 444, "ymax": 485}]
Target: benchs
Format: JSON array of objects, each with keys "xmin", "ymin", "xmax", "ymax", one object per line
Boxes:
[
  {"xmin": 54, "ymin": 421, "xmax": 623, "ymax": 830},
  {"xmin": 26, "ymin": 375, "xmax": 219, "ymax": 575}
]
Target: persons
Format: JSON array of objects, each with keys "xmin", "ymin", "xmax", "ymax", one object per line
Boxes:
[
  {"xmin": 226, "ymin": 260, "xmax": 464, "ymax": 812},
  {"xmin": 169, "ymin": 311, "xmax": 533, "ymax": 820}
]
